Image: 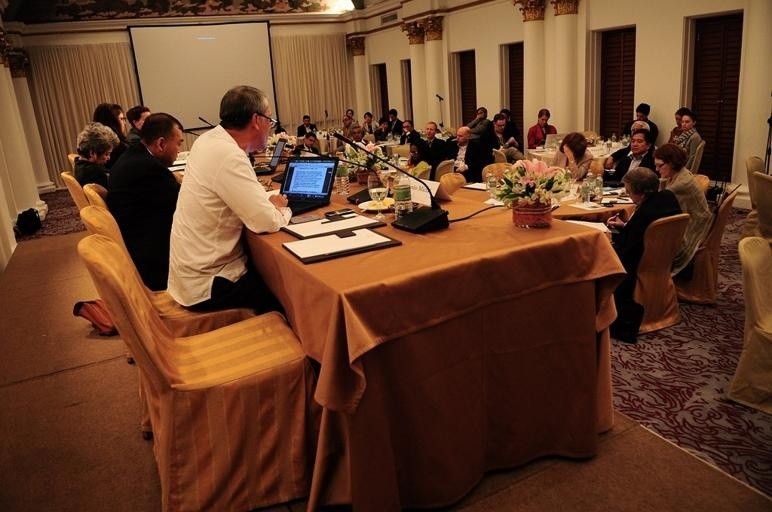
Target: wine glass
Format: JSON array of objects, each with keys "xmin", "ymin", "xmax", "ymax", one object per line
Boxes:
[{"xmin": 368, "ymin": 173, "xmax": 389, "ymax": 219}]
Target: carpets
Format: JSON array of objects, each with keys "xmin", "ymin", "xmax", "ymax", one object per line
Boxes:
[
  {"xmin": 604, "ymin": 202, "xmax": 771, "ymax": 511},
  {"xmin": 12, "ymin": 186, "xmax": 88, "ymax": 246}
]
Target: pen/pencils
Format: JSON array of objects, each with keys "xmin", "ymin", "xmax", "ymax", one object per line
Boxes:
[
  {"xmin": 551, "ymin": 203, "xmax": 560, "ymax": 211},
  {"xmin": 607, "ymin": 211, "xmax": 621, "ymax": 227}
]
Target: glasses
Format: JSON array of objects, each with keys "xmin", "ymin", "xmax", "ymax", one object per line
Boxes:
[
  {"xmin": 655, "ymin": 163, "xmax": 664, "ymax": 168},
  {"xmin": 256, "ymin": 109, "xmax": 279, "ymax": 129}
]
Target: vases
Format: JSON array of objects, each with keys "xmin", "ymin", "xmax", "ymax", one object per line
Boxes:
[
  {"xmin": 356, "ymin": 168, "xmax": 383, "ymax": 185},
  {"xmin": 273, "ymin": 149, "xmax": 289, "ymax": 162},
  {"xmin": 510, "ymin": 196, "xmax": 560, "ymax": 230}
]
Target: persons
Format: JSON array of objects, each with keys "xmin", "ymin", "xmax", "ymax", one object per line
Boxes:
[
  {"xmin": 527, "ymin": 109, "xmax": 557, "ymax": 148},
  {"xmin": 341, "ymin": 109, "xmax": 419, "ymax": 144},
  {"xmin": 604, "ymin": 102, "xmax": 705, "ymax": 343},
  {"xmin": 126, "ymin": 106, "xmax": 151, "ymax": 146},
  {"xmin": 553, "ymin": 133, "xmax": 592, "ymax": 180},
  {"xmin": 104, "ymin": 113, "xmax": 187, "ymax": 290},
  {"xmin": 413, "ymin": 108, "xmax": 520, "ymax": 183},
  {"xmin": 293, "ymin": 132, "xmax": 319, "ymax": 156},
  {"xmin": 167, "ymin": 85, "xmax": 297, "ymax": 316},
  {"xmin": 297, "ymin": 115, "xmax": 317, "ymax": 137},
  {"xmin": 94, "ymin": 103, "xmax": 134, "ymax": 170},
  {"xmin": 75, "ymin": 122, "xmax": 120, "ymax": 190}
]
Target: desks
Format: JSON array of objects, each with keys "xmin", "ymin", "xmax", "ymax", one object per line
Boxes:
[
  {"xmin": 294, "ymin": 124, "xmax": 449, "ymax": 157},
  {"xmin": 167, "ymin": 150, "xmax": 631, "ymax": 510},
  {"xmin": 525, "ymin": 137, "xmax": 639, "ymax": 170}
]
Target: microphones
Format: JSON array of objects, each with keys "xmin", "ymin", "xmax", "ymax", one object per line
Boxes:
[
  {"xmin": 436, "ymin": 95, "xmax": 443, "ymax": 100},
  {"xmin": 325, "ymin": 110, "xmax": 328, "ymax": 117},
  {"xmin": 333, "ymin": 133, "xmax": 449, "ymax": 232},
  {"xmin": 199, "ymin": 117, "xmax": 215, "ymax": 126},
  {"xmin": 183, "ymin": 129, "xmax": 255, "ymax": 163},
  {"xmin": 284, "ymin": 144, "xmax": 393, "ymax": 204}
]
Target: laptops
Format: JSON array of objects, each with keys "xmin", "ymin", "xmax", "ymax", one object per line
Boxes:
[
  {"xmin": 254, "ymin": 138, "xmax": 288, "ymax": 175},
  {"xmin": 280, "ymin": 157, "xmax": 339, "ymax": 216},
  {"xmin": 540, "ymin": 134, "xmax": 568, "ymax": 149}
]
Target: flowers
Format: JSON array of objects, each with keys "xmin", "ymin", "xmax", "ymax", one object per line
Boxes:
[
  {"xmin": 267, "ymin": 131, "xmax": 297, "ymax": 147},
  {"xmin": 353, "ymin": 142, "xmax": 385, "ymax": 168},
  {"xmin": 494, "ymin": 156, "xmax": 568, "ymax": 206},
  {"xmin": 455, "ymin": 160, "xmax": 469, "ymax": 174}
]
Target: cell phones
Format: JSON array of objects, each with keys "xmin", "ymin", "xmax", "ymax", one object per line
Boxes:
[
  {"xmin": 324, "ymin": 208, "xmax": 354, "ymax": 217},
  {"xmin": 604, "ymin": 203, "xmax": 613, "ymax": 207}
]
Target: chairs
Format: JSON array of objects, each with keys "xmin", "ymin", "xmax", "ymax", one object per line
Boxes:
[
  {"xmin": 491, "ymin": 146, "xmax": 509, "ymax": 164},
  {"xmin": 479, "ymin": 161, "xmax": 515, "ymax": 186},
  {"xmin": 432, "ymin": 157, "xmax": 459, "ymax": 183},
  {"xmin": 437, "ymin": 170, "xmax": 469, "ymax": 202}
]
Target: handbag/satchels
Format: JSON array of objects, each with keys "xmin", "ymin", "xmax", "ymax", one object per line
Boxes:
[
  {"xmin": 706, "ymin": 176, "xmax": 726, "ymax": 214},
  {"xmin": 17, "ymin": 208, "xmax": 41, "ymax": 235},
  {"xmin": 73, "ymin": 298, "xmax": 117, "ymax": 336}
]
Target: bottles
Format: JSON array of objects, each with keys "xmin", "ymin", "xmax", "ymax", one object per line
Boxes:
[
  {"xmin": 562, "ymin": 170, "xmax": 603, "ymax": 206},
  {"xmin": 606, "ymin": 132, "xmax": 630, "ymax": 154},
  {"xmin": 392, "ymin": 166, "xmax": 413, "ymax": 220},
  {"xmin": 266, "ymin": 138, "xmax": 273, "ymax": 160},
  {"xmin": 387, "ymin": 131, "xmax": 393, "ymax": 143},
  {"xmin": 486, "ymin": 170, "xmax": 493, "ymax": 193},
  {"xmin": 489, "ymin": 176, "xmax": 496, "ymax": 199},
  {"xmin": 393, "ymin": 152, "xmax": 400, "ymax": 168},
  {"xmin": 335, "ymin": 152, "xmax": 350, "ymax": 196}
]
